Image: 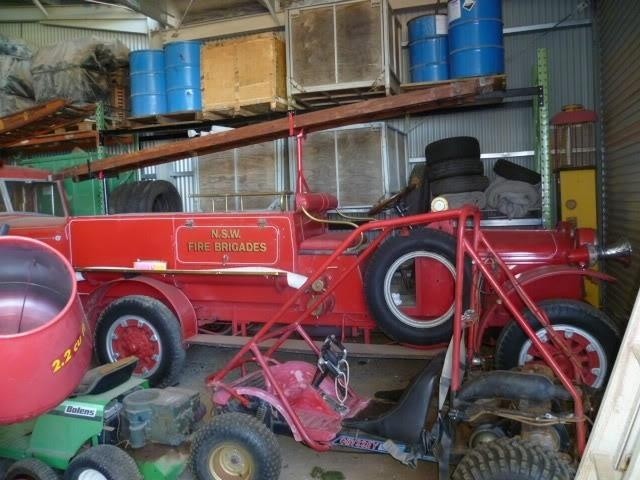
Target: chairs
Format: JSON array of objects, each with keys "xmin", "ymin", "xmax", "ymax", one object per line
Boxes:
[
  {"xmin": 339, "ymin": 348, "xmax": 448, "ymax": 445},
  {"xmin": 67, "ymin": 351, "xmax": 138, "ymax": 399}
]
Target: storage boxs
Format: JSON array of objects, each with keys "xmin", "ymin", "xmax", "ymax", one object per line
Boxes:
[
  {"xmin": 286, "ymin": 121, "xmax": 410, "ymax": 211},
  {"xmin": 196, "ymin": 134, "xmax": 290, "ymax": 214},
  {"xmin": 198, "ymin": 32, "xmax": 294, "ymax": 122},
  {"xmin": 284, "ymin": 0, "xmax": 405, "ymax": 111}
]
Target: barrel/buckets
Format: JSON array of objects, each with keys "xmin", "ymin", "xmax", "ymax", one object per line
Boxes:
[
  {"xmin": 161, "ymin": 40, "xmax": 203, "ymax": 112},
  {"xmin": 446, "ymin": 1, "xmax": 506, "ymax": 80},
  {"xmin": 407, "ymin": 13, "xmax": 445, "ymax": 82},
  {"xmin": 122, "ymin": 388, "xmax": 160, "ymax": 450},
  {"xmin": 128, "ymin": 49, "xmax": 162, "ymax": 115}
]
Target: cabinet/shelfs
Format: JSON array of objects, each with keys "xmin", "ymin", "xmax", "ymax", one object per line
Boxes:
[{"xmin": 0, "ymin": 45, "xmax": 561, "ymax": 361}]
